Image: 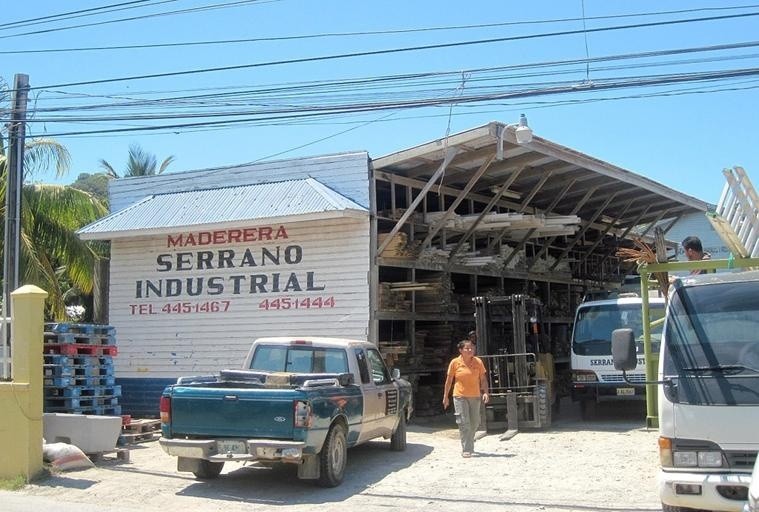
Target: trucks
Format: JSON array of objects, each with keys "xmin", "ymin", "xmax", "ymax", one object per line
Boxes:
[
  {"xmin": 604, "ymin": 277, "xmax": 759, "ymax": 511},
  {"xmin": 571, "ymin": 294, "xmax": 667, "ymax": 418}
]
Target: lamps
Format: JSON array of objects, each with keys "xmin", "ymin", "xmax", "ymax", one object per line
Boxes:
[{"xmin": 497, "ymin": 113, "xmax": 534, "ymax": 160}]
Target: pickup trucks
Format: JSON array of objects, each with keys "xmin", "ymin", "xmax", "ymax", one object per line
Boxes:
[{"xmin": 158, "ymin": 335, "xmax": 415, "ymax": 489}]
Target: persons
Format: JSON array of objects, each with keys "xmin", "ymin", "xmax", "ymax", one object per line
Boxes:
[
  {"xmin": 681, "ymin": 236, "xmax": 717, "ymax": 275},
  {"xmin": 442, "ymin": 339, "xmax": 490, "ymax": 459},
  {"xmin": 468, "ymin": 331, "xmax": 477, "ymax": 346}
]
